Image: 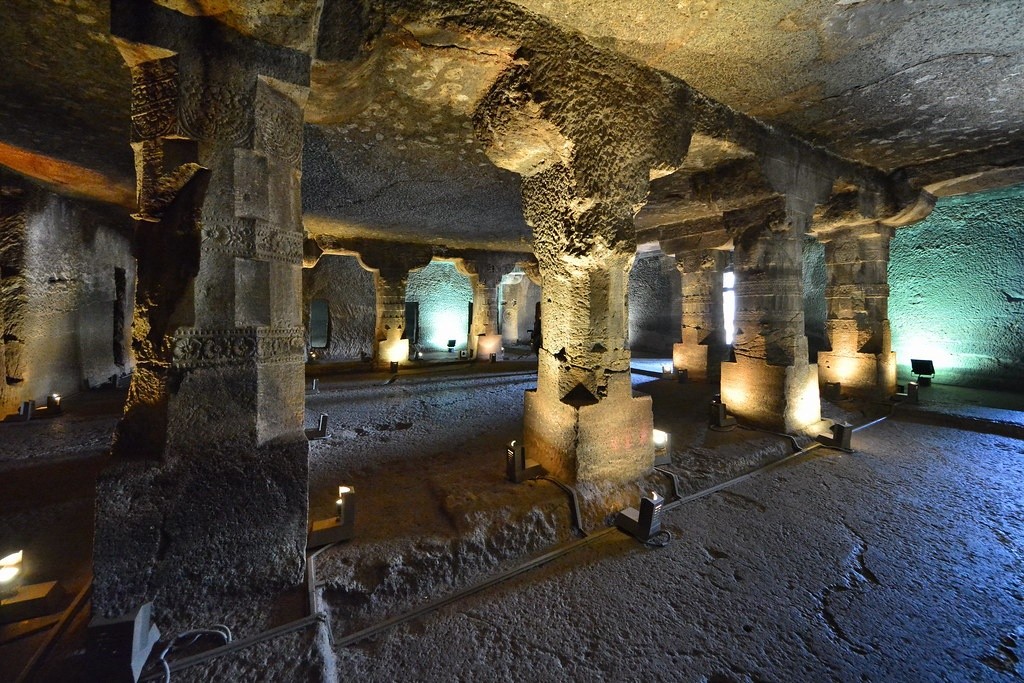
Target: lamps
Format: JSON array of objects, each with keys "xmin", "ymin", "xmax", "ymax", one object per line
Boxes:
[
  {"xmin": 35, "ymin": 392, "xmax": 64, "ymax": 418},
  {"xmin": 447, "ymin": 340, "xmax": 456, "ymax": 352},
  {"xmin": 825, "ymin": 381, "xmax": 842, "ymax": 398},
  {"xmin": 305, "ymin": 378, "xmax": 319, "ymax": 395},
  {"xmin": 652, "ymin": 428, "xmax": 672, "ymax": 466},
  {"xmin": 0, "ymin": 547, "xmax": 25, "ymax": 599},
  {"xmin": 709, "ymin": 401, "xmax": 737, "ymax": 428},
  {"xmin": 911, "ymin": 359, "xmax": 936, "ymax": 387},
  {"xmin": 86, "ymin": 603, "xmax": 161, "ymax": 683},
  {"xmin": 614, "ymin": 490, "xmax": 671, "ymax": 546},
  {"xmin": 892, "ymin": 380, "xmax": 919, "ymax": 405},
  {"xmin": 307, "ymin": 486, "xmax": 357, "ymax": 549},
  {"xmin": 507, "ymin": 439, "xmax": 542, "ymax": 483},
  {"xmin": 389, "ymin": 361, "xmax": 398, "ymax": 373},
  {"xmin": 816, "ymin": 420, "xmax": 852, "ymax": 450},
  {"xmin": 311, "ymin": 413, "xmax": 329, "ymax": 439}
]
